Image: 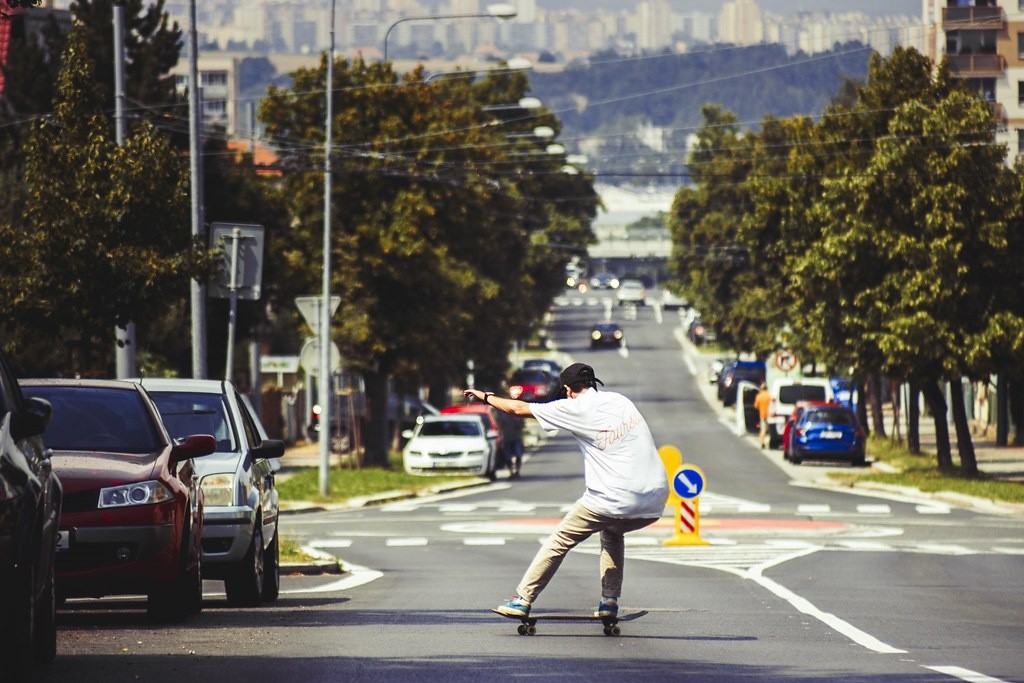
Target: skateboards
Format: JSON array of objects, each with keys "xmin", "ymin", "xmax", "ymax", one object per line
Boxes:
[{"xmin": 490, "ymin": 608, "xmax": 651, "ymax": 636}]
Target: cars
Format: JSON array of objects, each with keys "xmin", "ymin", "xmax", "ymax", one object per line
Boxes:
[
  {"xmin": 565, "ymin": 262, "xmax": 646, "ymax": 306},
  {"xmin": 590, "ymin": 324, "xmax": 623, "ymax": 349},
  {"xmin": 0, "ymin": 351, "xmax": 64, "ymax": 683},
  {"xmin": 18, "ymin": 379, "xmax": 216, "ymax": 622},
  {"xmin": 403, "ymin": 352, "xmax": 568, "ymax": 482},
  {"xmin": 661, "ymin": 287, "xmax": 720, "ymax": 345},
  {"xmin": 709, "ymin": 353, "xmax": 867, "ymax": 467},
  {"xmin": 118, "ymin": 378, "xmax": 285, "ymax": 608}
]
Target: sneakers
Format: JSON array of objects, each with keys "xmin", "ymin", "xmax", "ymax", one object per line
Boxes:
[
  {"xmin": 593, "ymin": 599, "xmax": 618, "ymax": 617},
  {"xmin": 498, "ymin": 595, "xmax": 531, "ymax": 618}
]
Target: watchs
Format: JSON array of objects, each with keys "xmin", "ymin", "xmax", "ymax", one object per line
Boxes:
[{"xmin": 483, "ymin": 392, "xmax": 494, "ymax": 403}]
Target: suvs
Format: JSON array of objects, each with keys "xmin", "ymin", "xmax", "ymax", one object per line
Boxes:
[{"xmin": 307, "ymin": 384, "xmax": 444, "ymax": 456}]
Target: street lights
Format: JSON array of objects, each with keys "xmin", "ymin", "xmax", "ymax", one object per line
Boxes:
[{"xmin": 385, "ymin": 4, "xmax": 516, "ymax": 62}]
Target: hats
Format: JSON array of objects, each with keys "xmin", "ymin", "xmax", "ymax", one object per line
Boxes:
[{"xmin": 555, "ymin": 363, "xmax": 605, "ymax": 400}]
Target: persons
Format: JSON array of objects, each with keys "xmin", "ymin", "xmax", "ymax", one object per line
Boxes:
[
  {"xmin": 753, "ymin": 382, "xmax": 774, "ymax": 450},
  {"xmin": 463, "ymin": 363, "xmax": 669, "ymax": 618},
  {"xmin": 496, "ymin": 384, "xmax": 528, "ymax": 478}
]
